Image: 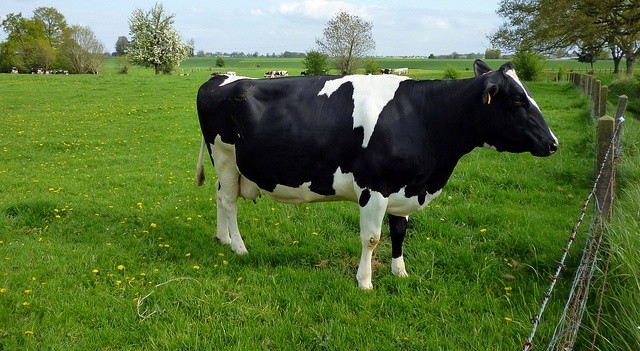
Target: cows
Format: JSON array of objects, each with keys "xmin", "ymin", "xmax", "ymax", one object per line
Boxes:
[
  {"xmin": 194, "ymin": 58, "xmax": 559, "ymax": 290},
  {"xmin": 301, "ymin": 71, "xmax": 305, "ymax": 75},
  {"xmin": 394, "ymin": 67, "xmax": 409, "ymax": 75},
  {"xmin": 380, "ymin": 67, "xmax": 392, "ymax": 74},
  {"xmin": 224, "ymin": 71, "xmax": 236, "ymax": 76},
  {"xmin": 270, "ymin": 70, "xmax": 282, "ymax": 77},
  {"xmin": 278, "ymin": 70, "xmax": 288, "ymax": 76},
  {"xmin": 264, "ymin": 71, "xmax": 272, "ymax": 76}
]
